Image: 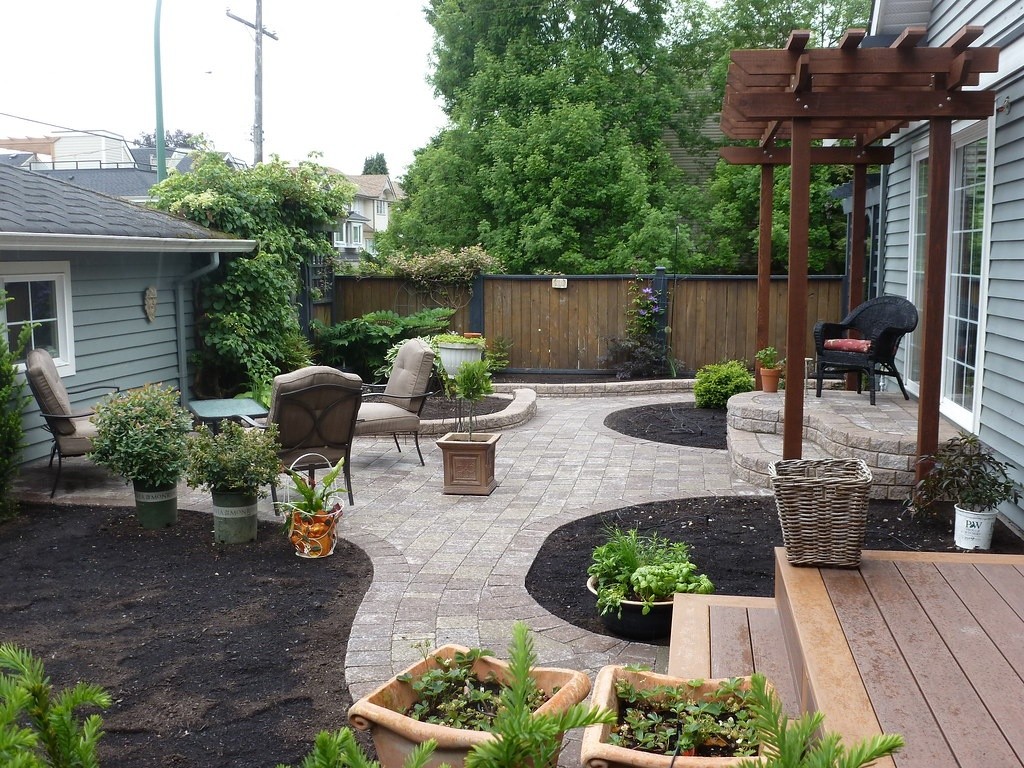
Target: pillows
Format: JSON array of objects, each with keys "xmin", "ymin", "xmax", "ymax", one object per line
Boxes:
[{"xmin": 824, "ymin": 339, "xmax": 872, "ymax": 353}]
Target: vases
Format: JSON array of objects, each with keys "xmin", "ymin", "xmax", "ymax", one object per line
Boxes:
[{"xmin": 633, "ymin": 345, "xmax": 649, "ymax": 365}]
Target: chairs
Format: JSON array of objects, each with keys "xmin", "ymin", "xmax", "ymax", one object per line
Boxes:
[
  {"xmin": 353, "ymin": 339, "xmax": 435, "ymax": 466},
  {"xmin": 227, "ymin": 366, "xmax": 362, "ymax": 517},
  {"xmin": 25, "ymin": 347, "xmax": 120, "ymax": 499},
  {"xmin": 813, "ymin": 295, "xmax": 918, "ymax": 405}
]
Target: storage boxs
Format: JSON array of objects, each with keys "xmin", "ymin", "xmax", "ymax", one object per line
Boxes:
[
  {"xmin": 842, "ymin": 327, "xmax": 865, "ymax": 339},
  {"xmin": 767, "ymin": 457, "xmax": 873, "ymax": 569}
]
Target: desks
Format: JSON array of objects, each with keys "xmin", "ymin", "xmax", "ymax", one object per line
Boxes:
[{"xmin": 188, "ymin": 399, "xmax": 269, "ymax": 443}]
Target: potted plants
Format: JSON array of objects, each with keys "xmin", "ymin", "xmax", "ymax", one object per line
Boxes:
[
  {"xmin": 754, "ymin": 346, "xmax": 782, "ymax": 392},
  {"xmin": 597, "ymin": 337, "xmax": 683, "ymax": 379},
  {"xmin": 348, "ymin": 638, "xmax": 592, "ymax": 768},
  {"xmin": 900, "ymin": 426, "xmax": 1024, "ymax": 550},
  {"xmin": 436, "ymin": 359, "xmax": 503, "ymax": 496},
  {"xmin": 436, "ymin": 332, "xmax": 486, "ymax": 379},
  {"xmin": 83, "ymin": 381, "xmax": 195, "ymax": 530},
  {"xmin": 181, "ymin": 420, "xmax": 283, "ymax": 545},
  {"xmin": 580, "ymin": 662, "xmax": 781, "ymax": 768},
  {"xmin": 586, "ymin": 512, "xmax": 715, "ymax": 641}
]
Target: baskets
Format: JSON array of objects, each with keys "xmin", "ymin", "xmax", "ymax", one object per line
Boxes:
[{"xmin": 771, "ymin": 456, "xmax": 875, "ymax": 569}]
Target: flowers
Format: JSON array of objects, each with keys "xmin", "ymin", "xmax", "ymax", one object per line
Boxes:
[{"xmin": 624, "ymin": 277, "xmax": 667, "ymax": 352}]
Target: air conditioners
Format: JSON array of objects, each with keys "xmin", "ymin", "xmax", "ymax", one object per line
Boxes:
[{"xmin": 277, "ymin": 456, "xmax": 352, "ymax": 559}]
